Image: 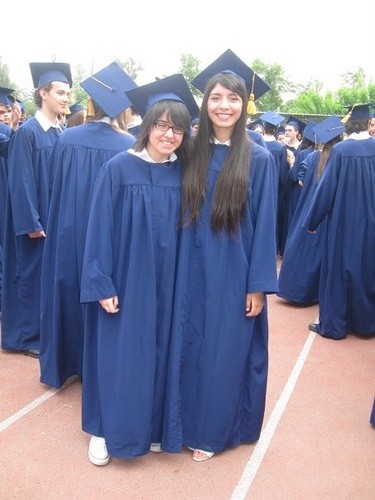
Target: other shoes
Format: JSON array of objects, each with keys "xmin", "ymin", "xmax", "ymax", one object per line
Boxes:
[{"xmin": 309, "ymin": 323, "xmax": 319, "ymax": 333}]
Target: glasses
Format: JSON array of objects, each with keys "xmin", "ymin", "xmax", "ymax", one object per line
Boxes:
[{"xmin": 152, "ymin": 118, "xmax": 185, "ymax": 134}]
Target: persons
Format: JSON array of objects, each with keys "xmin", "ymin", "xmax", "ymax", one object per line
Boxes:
[
  {"xmin": 0, "ymin": 62, "xmax": 142, "ymax": 389},
  {"xmin": 80, "ymin": 73, "xmax": 199, "ymax": 468},
  {"xmin": 160, "ymin": 50, "xmax": 279, "ymax": 461},
  {"xmin": 190, "ymin": 103, "xmax": 375, "ymax": 340}
]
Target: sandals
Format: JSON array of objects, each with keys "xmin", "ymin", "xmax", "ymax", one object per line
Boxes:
[{"xmin": 192, "ymin": 448, "xmax": 215, "ymax": 461}]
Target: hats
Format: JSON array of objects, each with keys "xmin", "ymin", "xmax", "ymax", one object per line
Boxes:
[
  {"xmin": 30, "ymin": 62, "xmax": 73, "ymax": 89},
  {"xmin": 246, "ymin": 110, "xmax": 317, "ymax": 150},
  {"xmin": 65, "ymin": 99, "xmax": 85, "ymax": 119},
  {"xmin": 314, "ymin": 115, "xmax": 348, "ymax": 143},
  {"xmin": 190, "ymin": 48, "xmax": 271, "ymax": 115},
  {"xmin": 126, "ymin": 74, "xmax": 200, "ymax": 121},
  {"xmin": 340, "ymin": 102, "xmax": 375, "ymax": 124},
  {"xmin": 0, "ymin": 86, "xmax": 26, "ymax": 115},
  {"xmin": 79, "ymin": 61, "xmax": 139, "ymax": 119},
  {"xmin": 189, "ymin": 114, "xmax": 200, "ymax": 128}
]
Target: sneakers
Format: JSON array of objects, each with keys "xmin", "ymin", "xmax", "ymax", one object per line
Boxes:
[{"xmin": 88, "ymin": 434, "xmax": 111, "ymax": 466}]
